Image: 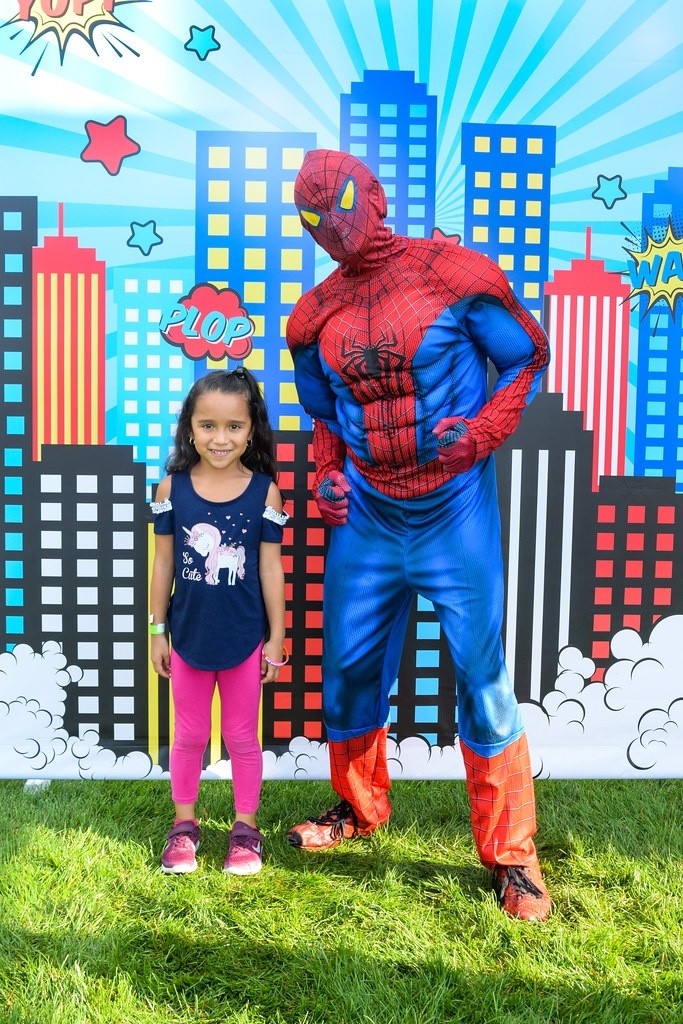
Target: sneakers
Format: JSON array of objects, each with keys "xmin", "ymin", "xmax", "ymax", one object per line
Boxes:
[
  {"xmin": 223, "ymin": 820, "xmax": 264, "ymax": 874},
  {"xmin": 493, "ymin": 858, "xmax": 551, "ymax": 922},
  {"xmin": 160, "ymin": 817, "xmax": 201, "ymax": 874},
  {"xmin": 287, "ymin": 798, "xmax": 367, "ymax": 849}
]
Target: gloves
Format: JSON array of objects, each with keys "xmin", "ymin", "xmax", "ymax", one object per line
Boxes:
[
  {"xmin": 432, "ymin": 417, "xmax": 476, "ymax": 473},
  {"xmin": 316, "ymin": 470, "xmax": 353, "ymax": 527}
]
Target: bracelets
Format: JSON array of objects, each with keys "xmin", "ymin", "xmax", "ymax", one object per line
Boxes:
[
  {"xmin": 261, "ymin": 641, "xmax": 289, "ymax": 666},
  {"xmin": 148, "ymin": 615, "xmax": 165, "ymax": 635}
]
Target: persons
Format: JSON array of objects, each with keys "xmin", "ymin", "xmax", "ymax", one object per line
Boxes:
[
  {"xmin": 286, "ymin": 148, "xmax": 555, "ymax": 922},
  {"xmin": 148, "ymin": 365, "xmax": 289, "ymax": 875}
]
